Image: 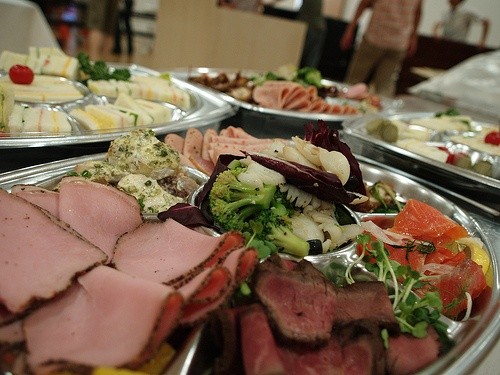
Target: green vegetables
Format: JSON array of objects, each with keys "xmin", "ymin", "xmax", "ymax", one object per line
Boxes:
[
  {"xmin": 344, "ymin": 234, "xmax": 444, "ymax": 349},
  {"xmin": 77, "ymin": 51, "xmax": 132, "ymax": 81}
]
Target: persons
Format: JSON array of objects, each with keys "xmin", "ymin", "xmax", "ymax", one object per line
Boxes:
[
  {"xmin": 341, "ymin": 0, "xmax": 423, "ymax": 95},
  {"xmin": 432, "ymin": 1, "xmax": 491, "ymax": 47},
  {"xmin": 104, "ymin": 0, "xmax": 134, "ymax": 57}
]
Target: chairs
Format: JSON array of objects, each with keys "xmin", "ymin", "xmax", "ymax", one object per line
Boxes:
[{"xmin": 157, "ymin": 0, "xmax": 308, "ymax": 80}]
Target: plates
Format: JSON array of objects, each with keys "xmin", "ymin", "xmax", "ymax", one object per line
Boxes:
[
  {"xmin": 0, "ymin": 151, "xmax": 500, "ymax": 375},
  {"xmin": 344, "ymin": 112, "xmax": 500, "ymax": 218},
  {"xmin": 167, "ymin": 64, "xmax": 410, "ymax": 122},
  {"xmin": 1, "ymin": 64, "xmax": 239, "ymax": 147}
]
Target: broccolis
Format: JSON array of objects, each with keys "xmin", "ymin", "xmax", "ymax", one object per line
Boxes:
[
  {"xmin": 237, "ymin": 202, "xmax": 310, "ymax": 258},
  {"xmin": 209, "ymin": 165, "xmax": 282, "ymax": 231}
]
